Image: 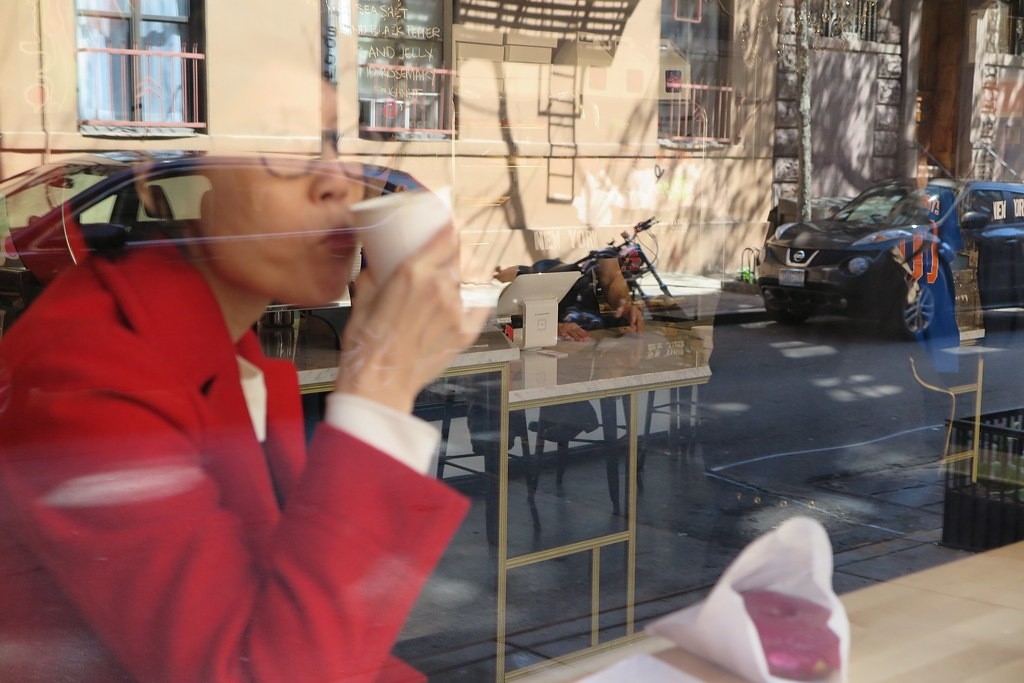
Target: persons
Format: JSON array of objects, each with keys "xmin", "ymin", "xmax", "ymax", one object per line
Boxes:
[{"xmin": 1, "ymin": 2, "xmax": 493, "ymax": 682}]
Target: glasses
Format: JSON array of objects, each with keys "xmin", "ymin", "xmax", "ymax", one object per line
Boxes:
[{"xmin": 211, "ymin": 130, "xmax": 398, "ymax": 179}]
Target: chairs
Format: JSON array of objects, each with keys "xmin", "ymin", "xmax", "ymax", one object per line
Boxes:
[{"xmin": 434, "ymin": 317, "xmax": 949, "ymax": 544}]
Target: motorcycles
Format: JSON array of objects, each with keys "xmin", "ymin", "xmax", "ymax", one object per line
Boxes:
[{"xmin": 492, "ymin": 213, "xmax": 705, "ymax": 383}]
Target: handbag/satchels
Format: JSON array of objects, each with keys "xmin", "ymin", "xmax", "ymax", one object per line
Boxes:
[{"xmin": 648, "ymin": 516, "xmax": 851, "ymax": 683}]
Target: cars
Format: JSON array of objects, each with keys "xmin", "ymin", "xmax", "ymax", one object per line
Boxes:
[
  {"xmin": 754, "ymin": 177, "xmax": 1024, "ymax": 342},
  {"xmin": 1, "ymin": 146, "xmax": 435, "ymax": 359}
]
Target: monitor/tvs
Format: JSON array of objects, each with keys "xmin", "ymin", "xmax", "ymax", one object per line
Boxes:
[{"xmin": 491, "ymin": 270, "xmax": 581, "ymax": 351}]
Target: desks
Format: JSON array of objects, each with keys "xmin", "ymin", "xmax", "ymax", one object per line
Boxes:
[{"xmin": 254, "ymin": 266, "xmax": 1024, "ymax": 683}]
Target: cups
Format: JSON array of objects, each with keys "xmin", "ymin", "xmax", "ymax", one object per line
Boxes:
[{"xmin": 347, "ymin": 183, "xmax": 453, "ymax": 286}]
